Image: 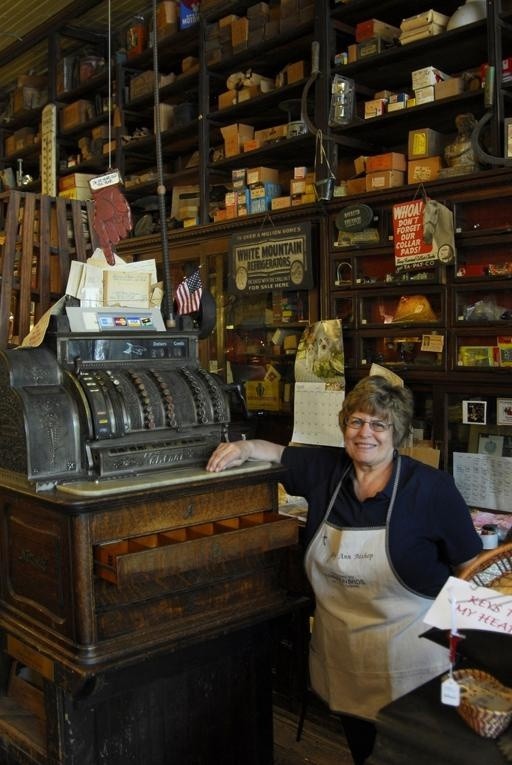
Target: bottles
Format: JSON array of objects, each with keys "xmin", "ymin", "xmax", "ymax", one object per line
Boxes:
[{"xmin": 480, "ymin": 524, "xmax": 498, "ymax": 549}]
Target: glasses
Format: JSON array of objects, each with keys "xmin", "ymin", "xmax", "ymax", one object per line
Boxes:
[{"xmin": 345, "ymin": 414, "xmax": 392, "ymax": 433}]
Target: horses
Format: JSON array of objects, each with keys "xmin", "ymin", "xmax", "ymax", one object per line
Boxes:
[{"xmin": 421, "ymin": 191, "xmax": 456, "ymax": 257}]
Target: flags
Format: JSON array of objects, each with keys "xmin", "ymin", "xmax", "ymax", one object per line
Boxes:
[{"xmin": 175, "ymin": 269, "xmax": 204, "ymax": 316}]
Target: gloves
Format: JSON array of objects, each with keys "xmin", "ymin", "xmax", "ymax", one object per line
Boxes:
[{"xmin": 88, "ymin": 169, "xmax": 132, "ymax": 265}]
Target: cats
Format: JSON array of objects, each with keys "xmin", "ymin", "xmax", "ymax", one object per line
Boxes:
[
  {"xmin": 305, "ymin": 343, "xmax": 317, "ymax": 370},
  {"xmin": 318, "ymin": 338, "xmax": 331, "ymax": 361}
]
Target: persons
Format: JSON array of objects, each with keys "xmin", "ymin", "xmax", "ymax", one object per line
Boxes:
[{"xmin": 205, "ymin": 374, "xmax": 480, "ymax": 765}]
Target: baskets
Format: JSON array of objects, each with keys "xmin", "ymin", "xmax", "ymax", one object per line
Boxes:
[
  {"xmin": 459, "ymin": 545, "xmax": 512, "ymax": 594},
  {"xmin": 441, "ymin": 669, "xmax": 512, "ymax": 740}
]
